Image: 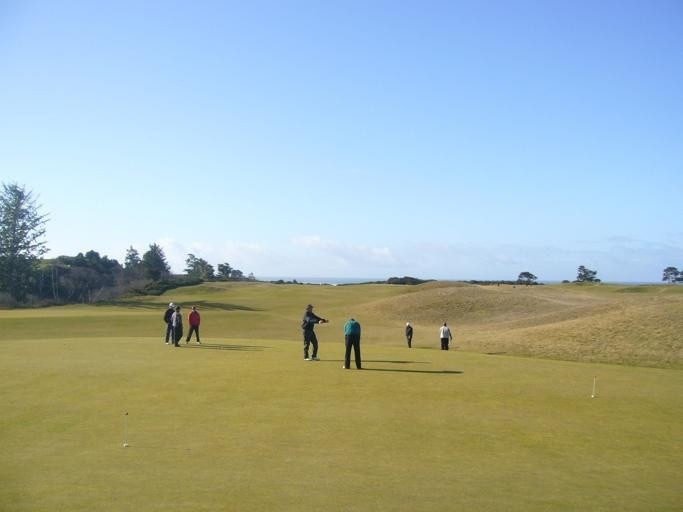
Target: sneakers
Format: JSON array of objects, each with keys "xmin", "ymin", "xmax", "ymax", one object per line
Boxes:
[
  {"xmin": 166, "ymin": 342, "xmax": 202, "ymax": 347},
  {"xmin": 305, "ymin": 357, "xmax": 321, "ymax": 360}
]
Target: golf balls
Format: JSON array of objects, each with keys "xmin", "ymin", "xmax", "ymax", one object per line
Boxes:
[{"xmin": 591, "ymin": 395, "xmax": 594, "ymax": 398}]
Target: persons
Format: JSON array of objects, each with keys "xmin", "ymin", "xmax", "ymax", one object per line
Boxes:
[
  {"xmin": 405, "ymin": 322, "xmax": 413, "ymax": 348},
  {"xmin": 300, "ymin": 304, "xmax": 329, "ymax": 361},
  {"xmin": 342, "ymin": 318, "xmax": 362, "ymax": 369},
  {"xmin": 439, "ymin": 322, "xmax": 452, "ymax": 350},
  {"xmin": 164, "ymin": 303, "xmax": 177, "ymax": 346},
  {"xmin": 185, "ymin": 306, "xmax": 202, "ymax": 344},
  {"xmin": 172, "ymin": 306, "xmax": 183, "ymax": 347}
]
